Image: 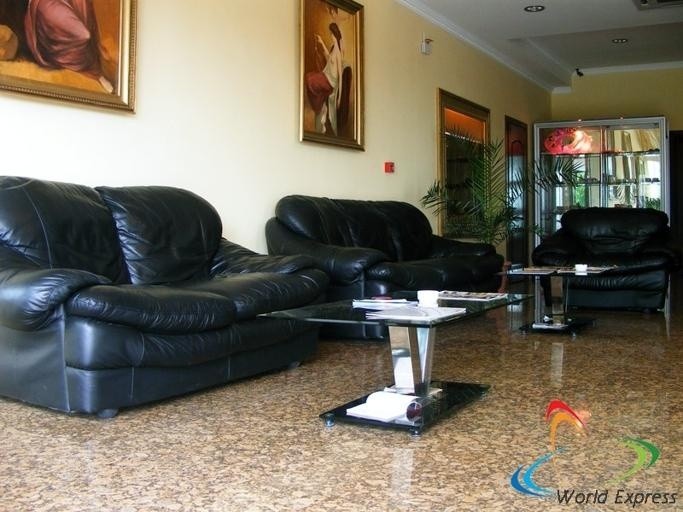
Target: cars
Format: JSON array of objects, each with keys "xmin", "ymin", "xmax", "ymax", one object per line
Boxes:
[
  {"xmin": 417, "ymin": 290, "xmax": 438, "ymax": 308},
  {"xmin": 575, "ymin": 264, "xmax": 587, "ymax": 272}
]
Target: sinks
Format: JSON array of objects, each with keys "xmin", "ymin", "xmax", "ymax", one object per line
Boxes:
[
  {"xmin": 0, "ymin": 174, "xmax": 330, "ymax": 418},
  {"xmin": 263, "ymin": 196, "xmax": 507, "ymax": 342}
]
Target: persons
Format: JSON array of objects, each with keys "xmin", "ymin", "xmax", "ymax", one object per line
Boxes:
[
  {"xmin": 305, "ymin": 22, "xmax": 342, "ymax": 134},
  {"xmin": 20, "ymin": 0, "xmax": 103, "ymax": 76}
]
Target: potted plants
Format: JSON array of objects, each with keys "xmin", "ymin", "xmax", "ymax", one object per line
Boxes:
[
  {"xmin": 297, "ymin": 1, "xmax": 365, "ymax": 152},
  {"xmin": 0, "ymin": 0, "xmax": 134, "ymax": 115}
]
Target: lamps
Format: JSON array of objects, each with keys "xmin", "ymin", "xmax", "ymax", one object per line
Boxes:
[{"xmin": 532, "ymin": 208, "xmax": 670, "ymax": 311}]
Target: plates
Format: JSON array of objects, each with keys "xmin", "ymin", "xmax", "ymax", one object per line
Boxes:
[{"xmin": 531, "ymin": 115, "xmax": 669, "ymax": 248}]
